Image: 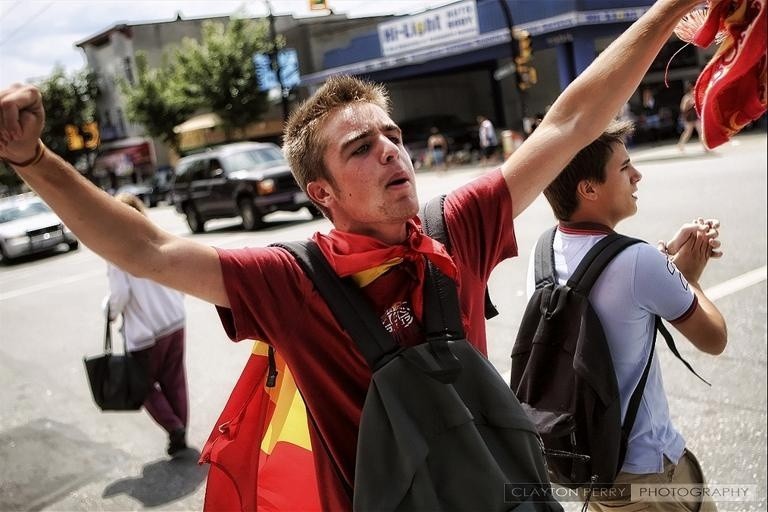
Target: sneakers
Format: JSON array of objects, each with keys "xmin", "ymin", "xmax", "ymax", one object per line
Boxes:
[{"xmin": 168, "ymin": 428, "xmax": 186, "ymax": 455}]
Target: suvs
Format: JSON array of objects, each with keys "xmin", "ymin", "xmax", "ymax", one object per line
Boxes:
[{"xmin": 172, "ymin": 142, "xmax": 324, "ymax": 232}]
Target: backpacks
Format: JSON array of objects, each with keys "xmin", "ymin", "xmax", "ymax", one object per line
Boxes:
[
  {"xmin": 510, "ymin": 224, "xmax": 661, "ymax": 491},
  {"xmin": 270, "ymin": 194, "xmax": 563, "ymax": 512}
]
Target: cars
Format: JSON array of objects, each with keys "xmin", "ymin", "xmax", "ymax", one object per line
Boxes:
[{"xmin": 0, "ymin": 194, "xmax": 77, "ymax": 258}]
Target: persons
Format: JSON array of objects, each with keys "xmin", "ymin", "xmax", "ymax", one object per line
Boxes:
[
  {"xmin": 529, "ymin": 118, "xmax": 727, "ymax": 512},
  {"xmin": 105, "ymin": 193, "xmax": 188, "ymax": 453},
  {"xmin": 0, "ymin": 0, "xmax": 710, "ymax": 512},
  {"xmin": 428, "ymin": 82, "xmax": 712, "ymax": 175}
]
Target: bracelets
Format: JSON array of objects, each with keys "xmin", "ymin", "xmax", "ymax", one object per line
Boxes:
[{"xmin": 2, "ymin": 142, "xmax": 40, "ymax": 167}]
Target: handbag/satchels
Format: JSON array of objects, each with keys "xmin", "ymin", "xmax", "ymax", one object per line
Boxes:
[{"xmin": 84, "ymin": 353, "xmax": 150, "ymax": 410}]
